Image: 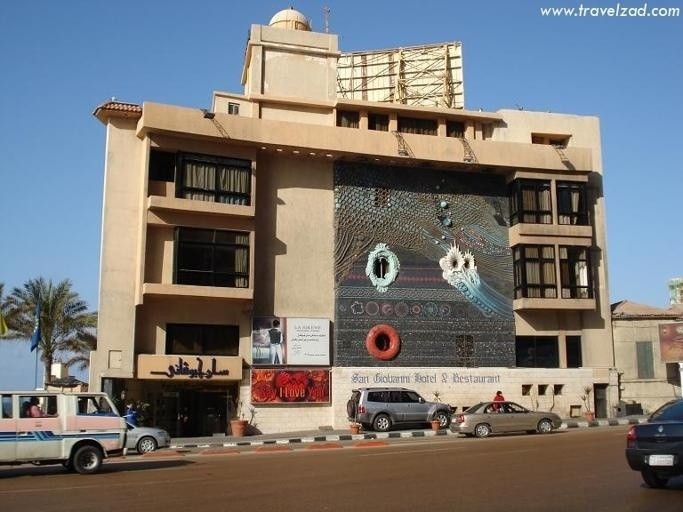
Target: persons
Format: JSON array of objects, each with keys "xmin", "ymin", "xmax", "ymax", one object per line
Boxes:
[
  {"xmin": 492, "ymin": 391, "xmax": 506, "ymax": 412},
  {"xmin": 265, "ymin": 319, "xmax": 284, "ymax": 366},
  {"xmin": 78, "ymin": 400, "xmax": 101, "ymax": 415},
  {"xmin": 21, "ymin": 401, "xmax": 30, "ymax": 417},
  {"xmin": 29, "ymin": 397, "xmax": 46, "ymax": 418}
]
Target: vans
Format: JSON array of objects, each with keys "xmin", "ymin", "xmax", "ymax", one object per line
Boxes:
[{"xmin": 0, "ymin": 390, "xmax": 127, "ymax": 474}]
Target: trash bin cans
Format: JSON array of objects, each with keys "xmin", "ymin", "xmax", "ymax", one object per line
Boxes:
[{"xmin": 616, "ymin": 400, "xmax": 626, "ymax": 416}]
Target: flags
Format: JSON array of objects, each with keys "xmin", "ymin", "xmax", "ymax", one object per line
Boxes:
[{"xmin": 27, "ymin": 297, "xmax": 44, "ymax": 353}]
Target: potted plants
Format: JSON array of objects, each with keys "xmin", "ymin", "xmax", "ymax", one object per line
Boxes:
[
  {"xmin": 431, "ymin": 392, "xmax": 441, "ymax": 431},
  {"xmin": 582, "ymin": 388, "xmax": 594, "ymax": 422}
]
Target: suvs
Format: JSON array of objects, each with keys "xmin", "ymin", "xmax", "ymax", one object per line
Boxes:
[{"xmin": 346, "ymin": 387, "xmax": 453, "ymax": 431}]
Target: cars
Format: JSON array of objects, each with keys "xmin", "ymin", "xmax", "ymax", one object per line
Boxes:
[
  {"xmin": 448, "ymin": 400, "xmax": 562, "ymax": 438},
  {"xmin": 124, "ymin": 420, "xmax": 170, "ymax": 454},
  {"xmin": 626, "ymin": 396, "xmax": 683, "ymax": 488}
]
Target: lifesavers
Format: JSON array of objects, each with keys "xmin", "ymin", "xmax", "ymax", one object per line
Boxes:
[{"xmin": 365, "ymin": 325, "xmax": 400, "ymax": 361}]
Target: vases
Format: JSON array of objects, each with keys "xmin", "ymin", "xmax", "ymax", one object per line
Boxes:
[
  {"xmin": 230, "ymin": 420, "xmax": 248, "ymax": 436},
  {"xmin": 350, "ymin": 425, "xmax": 360, "ymax": 435}
]
